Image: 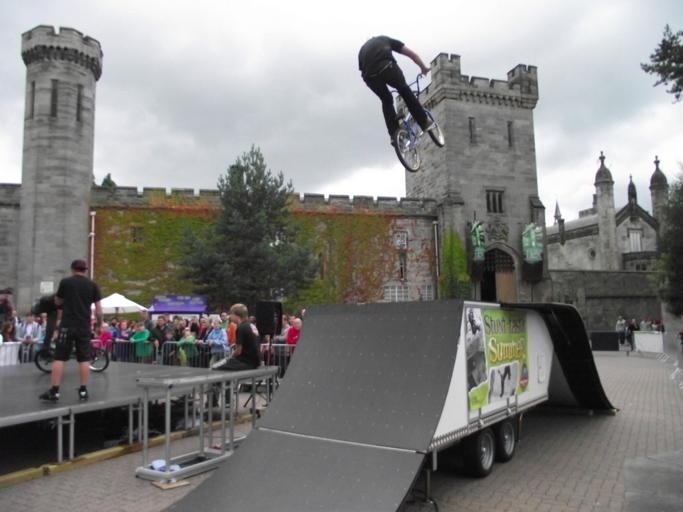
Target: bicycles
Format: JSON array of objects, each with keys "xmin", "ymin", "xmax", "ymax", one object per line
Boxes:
[
  {"xmin": 33, "ymin": 329, "xmax": 110, "ymax": 374},
  {"xmin": 383, "ymin": 67, "xmax": 445, "ymax": 174}
]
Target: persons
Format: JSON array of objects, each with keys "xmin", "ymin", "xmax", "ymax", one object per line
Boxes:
[
  {"xmin": 0, "ymin": 289, "xmax": 13, "ymax": 342},
  {"xmin": 205, "ymin": 303, "xmax": 261, "ymax": 408},
  {"xmin": 358, "ymin": 34, "xmax": 434, "ymax": 144},
  {"xmin": 615, "ymin": 315, "xmax": 664, "ymax": 344},
  {"xmin": 31, "ymin": 259, "xmax": 103, "ymax": 403},
  {"xmin": 13, "ymin": 309, "xmax": 306, "ymax": 377}
]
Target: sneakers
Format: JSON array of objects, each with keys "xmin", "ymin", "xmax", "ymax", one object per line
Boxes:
[
  {"xmin": 78, "ymin": 385, "xmax": 89, "ymax": 401},
  {"xmin": 39, "ymin": 390, "xmax": 61, "ymax": 402}
]
